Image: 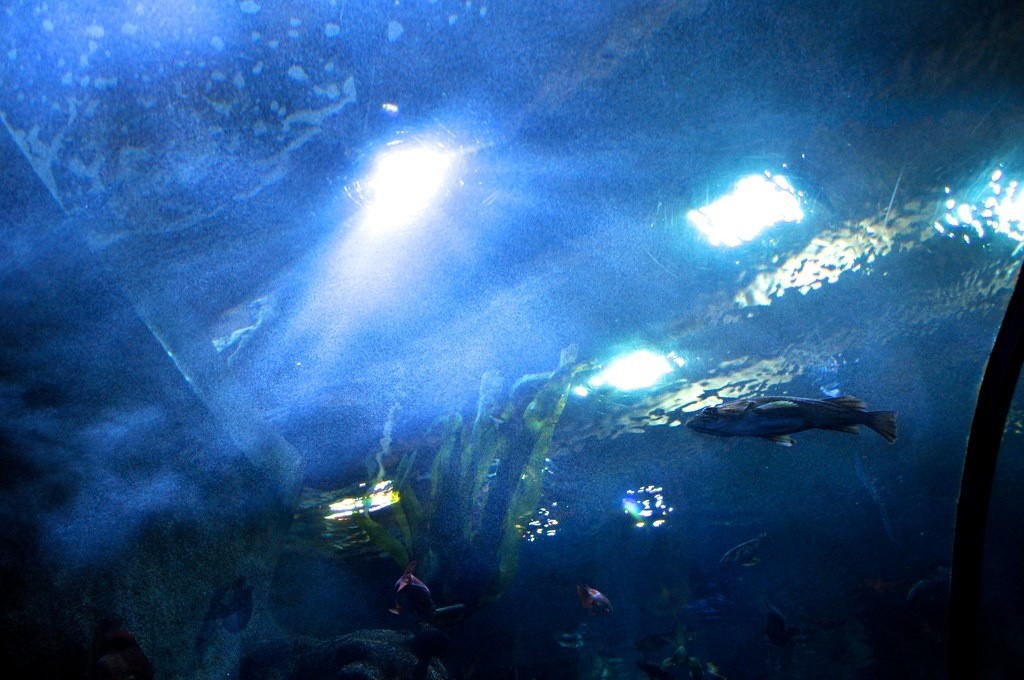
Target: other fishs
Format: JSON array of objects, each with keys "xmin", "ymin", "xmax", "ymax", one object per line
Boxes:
[
  {"xmin": 381, "ymin": 528, "xmax": 942, "ymax": 680},
  {"xmin": 683, "ymin": 395, "xmax": 900, "ymax": 451}
]
